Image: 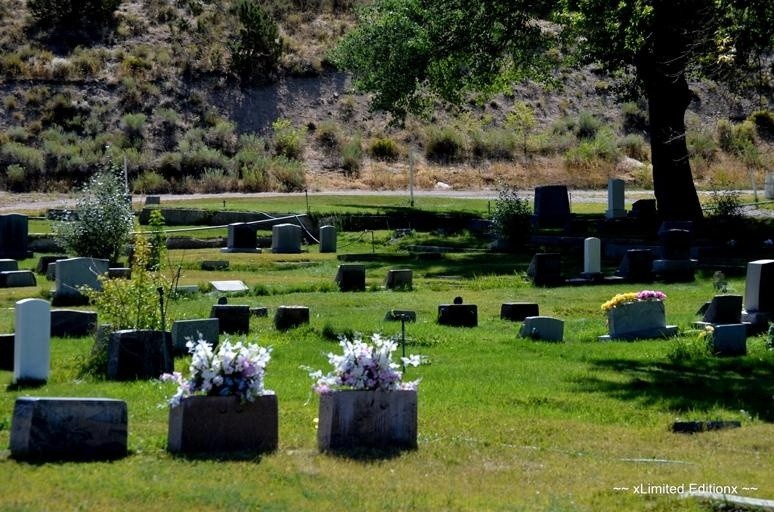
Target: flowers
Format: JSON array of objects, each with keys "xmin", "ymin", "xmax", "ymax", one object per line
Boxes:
[
  {"xmin": 47, "ymin": 143, "xmax": 136, "ymax": 259},
  {"xmin": 602, "ymin": 289, "xmax": 668, "ymax": 312},
  {"xmin": 298, "ymin": 331, "xmax": 425, "ymax": 397},
  {"xmin": 160, "ymin": 329, "xmax": 272, "ymax": 409}
]
